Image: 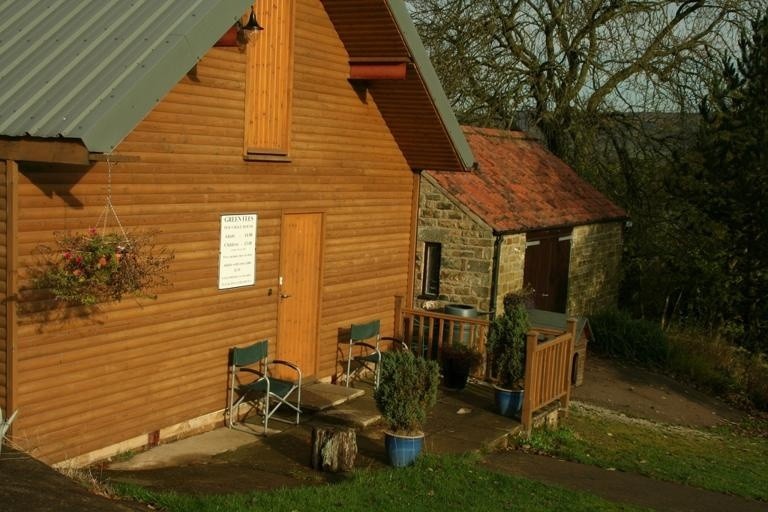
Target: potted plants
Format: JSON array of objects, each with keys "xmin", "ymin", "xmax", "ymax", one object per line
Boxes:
[
  {"xmin": 484, "ymin": 295, "xmax": 532, "ymax": 417},
  {"xmin": 374, "ymin": 347, "xmax": 442, "ymax": 468}
]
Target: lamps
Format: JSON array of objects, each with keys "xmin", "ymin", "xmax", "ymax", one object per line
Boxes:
[{"xmin": 624, "ymin": 218, "xmax": 634, "ymax": 234}]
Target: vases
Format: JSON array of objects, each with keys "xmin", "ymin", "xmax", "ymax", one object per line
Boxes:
[
  {"xmin": 71, "ymin": 249, "xmax": 130, "ymax": 285},
  {"xmin": 443, "ymin": 360, "xmax": 469, "ymax": 389}
]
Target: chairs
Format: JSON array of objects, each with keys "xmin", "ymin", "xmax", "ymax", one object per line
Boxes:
[
  {"xmin": 347, "ymin": 320, "xmax": 409, "ymax": 390},
  {"xmin": 228, "ymin": 339, "xmax": 301, "ymax": 434}
]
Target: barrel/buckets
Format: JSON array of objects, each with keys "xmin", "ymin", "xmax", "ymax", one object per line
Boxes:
[{"xmin": 444, "ymin": 302, "xmax": 479, "ymax": 350}]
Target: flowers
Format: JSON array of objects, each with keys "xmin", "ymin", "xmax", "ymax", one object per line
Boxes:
[
  {"xmin": 439, "ymin": 340, "xmax": 483, "ymax": 368},
  {"xmin": 23, "ymin": 226, "xmax": 175, "ymax": 312}
]
